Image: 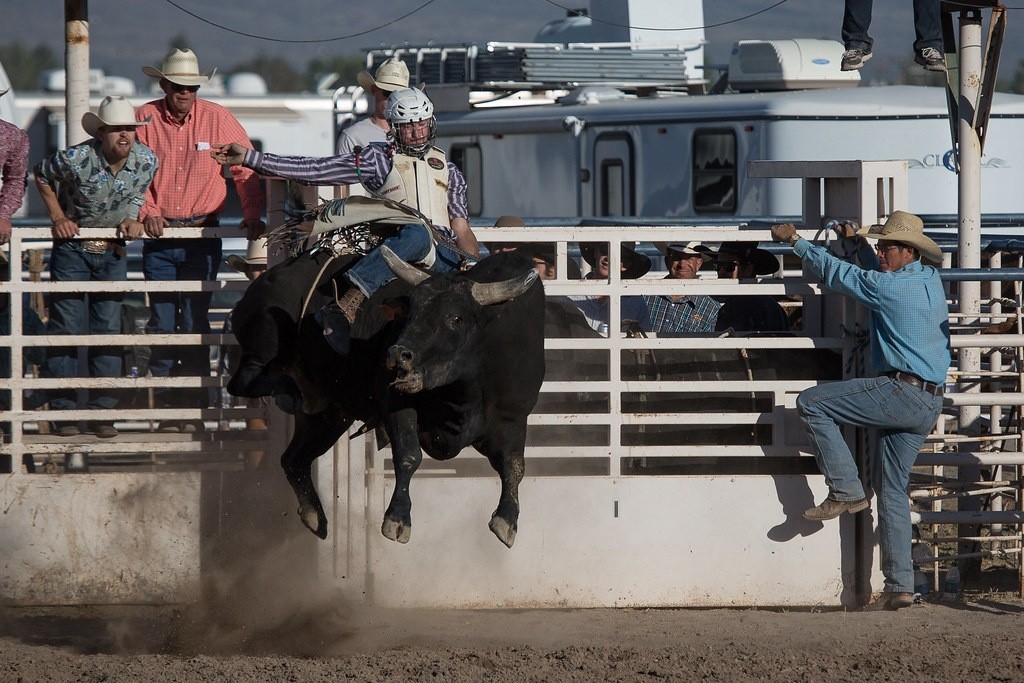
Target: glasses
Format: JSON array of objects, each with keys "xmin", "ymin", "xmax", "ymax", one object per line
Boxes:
[
  {"xmin": 164, "ymin": 79, "xmax": 201, "ymax": 93},
  {"xmin": 596, "ymin": 244, "xmax": 609, "ymax": 257},
  {"xmin": 374, "ymin": 85, "xmax": 391, "ymax": 99},
  {"xmin": 874, "ymin": 240, "xmax": 906, "ymax": 253},
  {"xmin": 711, "ymin": 259, "xmax": 750, "ymax": 272}
]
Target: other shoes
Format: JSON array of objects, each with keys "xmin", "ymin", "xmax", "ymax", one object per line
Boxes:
[
  {"xmin": 86, "ymin": 419, "xmax": 117, "ymax": 437},
  {"xmin": 158, "ymin": 420, "xmax": 180, "ymax": 434},
  {"xmin": 313, "ymin": 296, "xmax": 351, "ymax": 355},
  {"xmin": 181, "ymin": 419, "xmax": 204, "ymax": 434},
  {"xmin": 57, "ymin": 419, "xmax": 78, "ymax": 435}
]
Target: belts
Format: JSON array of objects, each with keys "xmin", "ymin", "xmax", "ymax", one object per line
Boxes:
[
  {"xmin": 877, "ymin": 368, "xmax": 945, "ymax": 397},
  {"xmin": 169, "ymin": 218, "xmax": 217, "ymax": 227},
  {"xmin": 79, "ymin": 238, "xmax": 125, "ymax": 257}
]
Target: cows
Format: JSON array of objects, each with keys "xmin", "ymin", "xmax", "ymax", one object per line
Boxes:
[{"xmin": 225, "ymin": 245, "xmax": 547, "ymax": 549}]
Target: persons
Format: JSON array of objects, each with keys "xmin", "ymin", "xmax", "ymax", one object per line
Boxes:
[
  {"xmin": 1, "ymin": 97, "xmax": 159, "ymax": 436},
  {"xmin": 209, "ymin": 87, "xmax": 479, "ymax": 332},
  {"xmin": 133, "ymin": 48, "xmax": 266, "ymax": 429},
  {"xmin": 840, "ymin": 0, "xmax": 946, "ymax": 72},
  {"xmin": 225, "ymin": 235, "xmax": 270, "ymax": 429},
  {"xmin": 770, "ymin": 210, "xmax": 951, "ymax": 609},
  {"xmin": 483, "ymin": 216, "xmax": 805, "ymax": 332},
  {"xmin": 335, "ymin": 58, "xmax": 426, "ymax": 255}
]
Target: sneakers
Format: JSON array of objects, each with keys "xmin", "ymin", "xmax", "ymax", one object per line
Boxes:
[
  {"xmin": 914, "ymin": 46, "xmax": 947, "ymax": 73},
  {"xmin": 803, "ymin": 495, "xmax": 869, "ymax": 520},
  {"xmin": 841, "ymin": 45, "xmax": 872, "ymax": 72},
  {"xmin": 879, "ymin": 590, "xmax": 914, "ymax": 611}
]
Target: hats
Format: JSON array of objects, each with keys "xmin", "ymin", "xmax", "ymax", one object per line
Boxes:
[
  {"xmin": 0, "ymin": 88, "xmax": 11, "ymax": 96},
  {"xmin": 693, "ymin": 238, "xmax": 780, "ymax": 275},
  {"xmin": 82, "ymin": 95, "xmax": 152, "ymax": 138},
  {"xmin": 357, "ymin": 59, "xmax": 425, "ymax": 94},
  {"xmin": 653, "ymin": 242, "xmax": 712, "ymax": 263},
  {"xmin": 856, "ymin": 211, "xmax": 943, "ymax": 264},
  {"xmin": 142, "ymin": 47, "xmax": 217, "ymax": 86},
  {"xmin": 481, "ymin": 217, "xmax": 528, "ymax": 254},
  {"xmin": 580, "ymin": 239, "xmax": 652, "ymax": 280}
]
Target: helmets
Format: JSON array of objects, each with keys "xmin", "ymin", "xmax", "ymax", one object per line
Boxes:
[{"xmin": 383, "ymin": 87, "xmax": 437, "ymax": 158}]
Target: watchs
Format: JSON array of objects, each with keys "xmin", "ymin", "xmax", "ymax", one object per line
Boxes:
[{"xmin": 789, "ymin": 233, "xmax": 801, "ymax": 247}]
